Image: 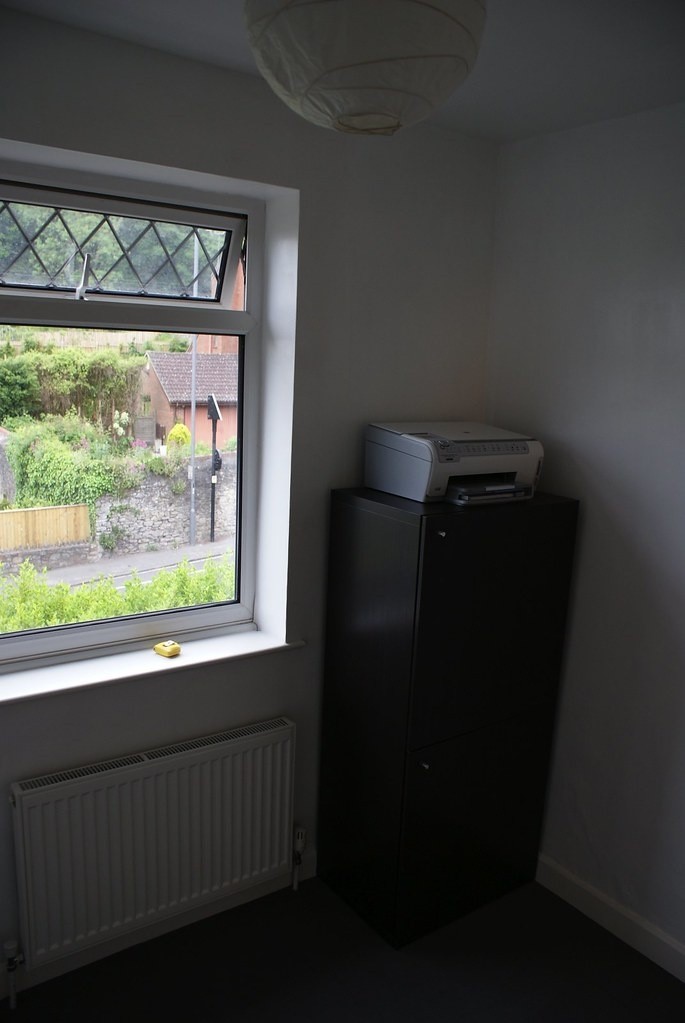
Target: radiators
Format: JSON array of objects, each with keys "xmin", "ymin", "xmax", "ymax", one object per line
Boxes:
[{"xmin": 8, "ymin": 716, "xmax": 294, "ymax": 973}]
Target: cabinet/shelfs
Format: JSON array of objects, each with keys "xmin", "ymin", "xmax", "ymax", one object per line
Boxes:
[{"xmin": 314, "ymin": 488, "xmax": 581, "ymax": 955}]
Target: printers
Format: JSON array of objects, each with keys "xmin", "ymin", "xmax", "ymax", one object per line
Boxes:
[{"xmin": 361, "ymin": 420, "xmax": 545, "ymax": 508}]
